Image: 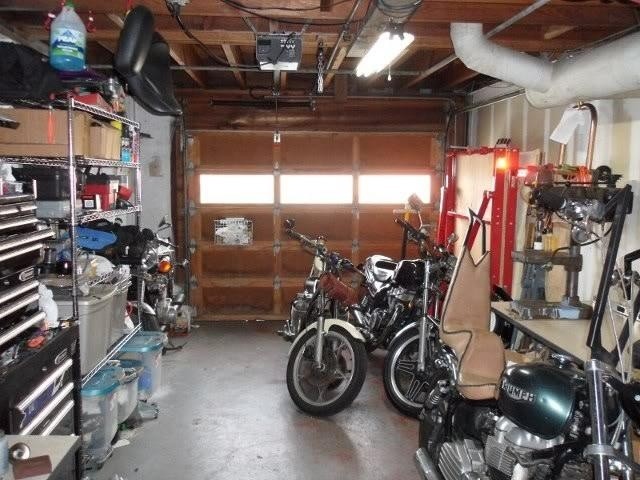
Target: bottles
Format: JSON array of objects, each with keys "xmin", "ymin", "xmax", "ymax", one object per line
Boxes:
[{"xmin": 0, "ymin": 429, "xmax": 10, "ymax": 477}]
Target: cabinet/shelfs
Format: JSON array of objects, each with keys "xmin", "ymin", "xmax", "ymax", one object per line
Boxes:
[
  {"xmin": 0, "ymin": 92, "xmax": 145, "ymax": 480},
  {"xmin": 180, "ymin": 126, "xmax": 444, "ymax": 325}
]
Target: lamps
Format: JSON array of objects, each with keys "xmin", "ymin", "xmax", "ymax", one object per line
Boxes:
[{"xmin": 350, "ymin": 25, "xmax": 416, "ymax": 80}]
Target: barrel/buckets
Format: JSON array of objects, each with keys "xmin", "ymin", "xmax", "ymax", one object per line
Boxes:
[{"xmin": 49, "ymin": 0, "xmax": 87, "ymax": 73}]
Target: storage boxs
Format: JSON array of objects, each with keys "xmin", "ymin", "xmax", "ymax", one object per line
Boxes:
[
  {"xmin": 0, "ymin": 104, "xmax": 91, "ymax": 159},
  {"xmin": 46, "ymin": 275, "xmax": 169, "ymax": 455},
  {"xmin": 90, "ymin": 116, "xmax": 123, "ymax": 161}
]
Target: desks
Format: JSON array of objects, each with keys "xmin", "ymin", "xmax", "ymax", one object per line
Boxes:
[
  {"xmin": 488, "ymin": 297, "xmax": 634, "ymax": 379},
  {"xmin": 0, "ymin": 434, "xmax": 82, "ymax": 480}
]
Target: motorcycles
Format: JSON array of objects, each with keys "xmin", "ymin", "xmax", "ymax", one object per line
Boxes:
[
  {"xmin": 111, "ymin": 215, "xmax": 191, "ymax": 356},
  {"xmin": 353, "ymin": 194, "xmax": 465, "ymax": 421},
  {"xmin": 411, "ymin": 190, "xmax": 640, "ymax": 479},
  {"xmin": 274, "ymin": 218, "xmax": 371, "ymax": 416}
]
